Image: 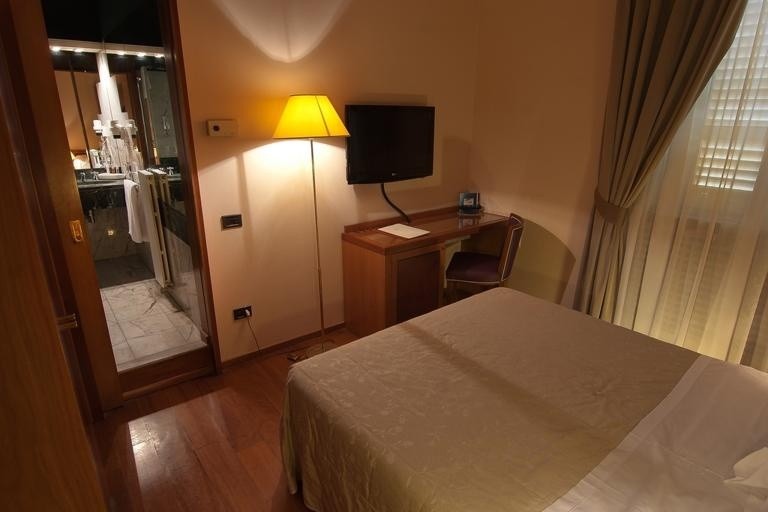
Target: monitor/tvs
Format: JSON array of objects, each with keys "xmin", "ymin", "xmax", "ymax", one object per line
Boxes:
[{"xmin": 346, "ymin": 104, "xmax": 435, "ymax": 184}]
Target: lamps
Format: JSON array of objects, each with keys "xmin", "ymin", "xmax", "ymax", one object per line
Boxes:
[{"xmin": 270, "ymin": 96, "xmax": 351, "ymax": 353}]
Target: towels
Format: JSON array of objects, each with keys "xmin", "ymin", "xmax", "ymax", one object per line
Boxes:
[{"xmin": 123, "ymin": 179, "xmax": 141, "ymax": 244}]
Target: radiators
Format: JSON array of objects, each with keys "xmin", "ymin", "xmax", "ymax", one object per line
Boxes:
[{"xmin": 138, "ymin": 170, "xmax": 172, "ymax": 290}]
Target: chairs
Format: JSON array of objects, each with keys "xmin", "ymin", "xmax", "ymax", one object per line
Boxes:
[{"xmin": 447, "ymin": 212, "xmax": 524, "ymax": 305}]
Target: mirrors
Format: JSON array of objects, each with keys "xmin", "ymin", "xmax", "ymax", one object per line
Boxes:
[{"xmin": 127, "ymin": 56, "xmax": 191, "ymax": 246}]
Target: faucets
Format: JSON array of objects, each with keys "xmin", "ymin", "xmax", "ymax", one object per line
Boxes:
[
  {"xmin": 167, "ymin": 166, "xmax": 175, "ymax": 175},
  {"xmin": 79, "ymin": 170, "xmax": 87, "ymax": 181}
]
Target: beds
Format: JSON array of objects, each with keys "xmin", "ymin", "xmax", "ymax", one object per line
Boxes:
[{"xmin": 288, "ymin": 286, "xmax": 767, "ymax": 511}]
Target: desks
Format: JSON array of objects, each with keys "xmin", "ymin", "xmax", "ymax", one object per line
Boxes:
[{"xmin": 342, "ymin": 206, "xmax": 507, "ymax": 338}]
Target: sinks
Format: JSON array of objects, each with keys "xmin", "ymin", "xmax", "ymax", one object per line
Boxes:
[
  {"xmin": 168, "ymin": 175, "xmax": 181, "ymax": 179},
  {"xmin": 77, "ymin": 180, "xmax": 116, "ymax": 186}
]
